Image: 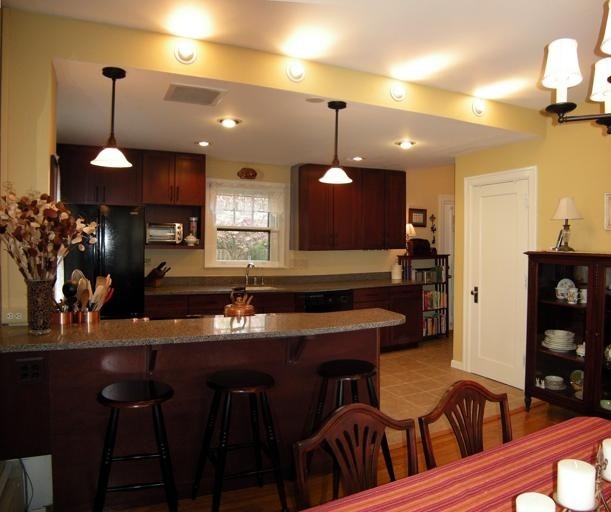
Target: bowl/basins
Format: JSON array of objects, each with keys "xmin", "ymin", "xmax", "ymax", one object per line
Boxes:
[{"xmin": 599, "ymin": 400, "xmax": 611, "ymax": 411}]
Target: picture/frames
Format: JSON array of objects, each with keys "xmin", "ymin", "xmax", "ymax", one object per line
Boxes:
[
  {"xmin": 409, "ymin": 208, "xmax": 427, "ymax": 227},
  {"xmin": 603, "ymin": 192, "xmax": 611, "ymax": 230}
]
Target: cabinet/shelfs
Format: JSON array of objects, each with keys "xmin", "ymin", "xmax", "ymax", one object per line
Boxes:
[
  {"xmin": 354, "ymin": 285, "xmax": 423, "ymax": 347},
  {"xmin": 142, "ymin": 149, "xmax": 206, "ymax": 250},
  {"xmin": 144, "ymin": 294, "xmax": 231, "ymax": 319},
  {"xmin": 248, "ymin": 293, "xmax": 295, "ymax": 313},
  {"xmin": 398, "ymin": 254, "xmax": 450, "ymax": 336},
  {"xmin": 523, "ymin": 250, "xmax": 611, "ymax": 419},
  {"xmin": 290, "ymin": 164, "xmax": 406, "ymax": 251},
  {"xmin": 56, "ymin": 143, "xmax": 142, "ymax": 206}
]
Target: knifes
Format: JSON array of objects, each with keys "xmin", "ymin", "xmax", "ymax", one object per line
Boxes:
[{"xmin": 156, "ymin": 262, "xmax": 171, "ymax": 277}]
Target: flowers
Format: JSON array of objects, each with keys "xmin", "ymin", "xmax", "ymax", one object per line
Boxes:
[{"xmin": 0, "ymin": 185, "xmax": 98, "ymax": 281}]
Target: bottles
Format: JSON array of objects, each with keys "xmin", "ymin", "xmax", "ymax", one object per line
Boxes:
[
  {"xmin": 390, "ymin": 256, "xmax": 403, "ymax": 279},
  {"xmin": 189, "ymin": 217, "xmax": 198, "ymax": 237}
]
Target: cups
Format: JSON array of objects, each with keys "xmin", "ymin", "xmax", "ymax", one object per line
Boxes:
[
  {"xmin": 564, "ymin": 288, "xmax": 578, "ymax": 304},
  {"xmin": 555, "ymin": 288, "xmax": 565, "ymax": 299},
  {"xmin": 51, "ymin": 311, "xmax": 74, "ymax": 324},
  {"xmin": 577, "ymin": 288, "xmax": 587, "ymax": 304},
  {"xmin": 544, "ymin": 375, "xmax": 564, "ymax": 387},
  {"xmin": 77, "ymin": 311, "xmax": 101, "ymax": 323}
]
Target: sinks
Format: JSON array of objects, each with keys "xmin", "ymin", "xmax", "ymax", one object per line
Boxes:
[{"xmin": 244, "ymin": 285, "xmax": 276, "ymax": 291}]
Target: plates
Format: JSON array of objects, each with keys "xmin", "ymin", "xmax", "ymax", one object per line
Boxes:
[
  {"xmin": 574, "ymin": 390, "xmax": 583, "ymax": 400},
  {"xmin": 570, "ymin": 369, "xmax": 584, "ymax": 390},
  {"xmin": 541, "ymin": 328, "xmax": 577, "ymax": 353},
  {"xmin": 557, "ymin": 278, "xmax": 575, "ymax": 299},
  {"xmin": 546, "ymin": 385, "xmax": 567, "ymax": 391}
]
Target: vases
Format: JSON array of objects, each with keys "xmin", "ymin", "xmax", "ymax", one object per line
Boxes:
[{"xmin": 27, "ymin": 281, "xmax": 53, "ymax": 336}]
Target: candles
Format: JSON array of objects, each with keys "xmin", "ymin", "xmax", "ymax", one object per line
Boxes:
[{"xmin": 515, "ymin": 437, "xmax": 611, "ymax": 511}]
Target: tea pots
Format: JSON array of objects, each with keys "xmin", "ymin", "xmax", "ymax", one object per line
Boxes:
[
  {"xmin": 184, "ymin": 233, "xmax": 204, "ymax": 246},
  {"xmin": 223, "ymin": 287, "xmax": 255, "ymax": 317}
]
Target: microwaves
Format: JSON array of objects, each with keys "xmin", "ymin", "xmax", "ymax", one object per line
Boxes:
[{"xmin": 145, "ymin": 221, "xmax": 184, "ymax": 245}]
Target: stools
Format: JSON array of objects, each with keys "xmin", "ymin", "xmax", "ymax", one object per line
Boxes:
[
  {"xmin": 95, "ymin": 380, "xmax": 175, "ymax": 512},
  {"xmin": 306, "ymin": 359, "xmax": 395, "ymax": 497},
  {"xmin": 192, "ymin": 369, "xmax": 288, "ymax": 511}
]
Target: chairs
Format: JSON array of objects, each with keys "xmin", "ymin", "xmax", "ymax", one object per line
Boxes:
[
  {"xmin": 293, "ymin": 402, "xmax": 419, "ymax": 509},
  {"xmin": 419, "ymin": 379, "xmax": 513, "ymax": 471}
]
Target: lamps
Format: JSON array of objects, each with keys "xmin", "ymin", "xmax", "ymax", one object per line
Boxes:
[
  {"xmin": 90, "ymin": 67, "xmax": 134, "ymax": 167},
  {"xmin": 319, "ymin": 101, "xmax": 354, "ymax": 185},
  {"xmin": 551, "ymin": 197, "xmax": 584, "ymax": 251},
  {"xmin": 350, "ymin": 154, "xmax": 368, "ymax": 168},
  {"xmin": 406, "ymin": 223, "xmax": 416, "ymax": 256},
  {"xmin": 541, "ymin": 0, "xmax": 611, "ymax": 134}
]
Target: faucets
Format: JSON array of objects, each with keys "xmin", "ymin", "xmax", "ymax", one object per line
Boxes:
[{"xmin": 245, "ymin": 263, "xmax": 255, "ymax": 284}]
